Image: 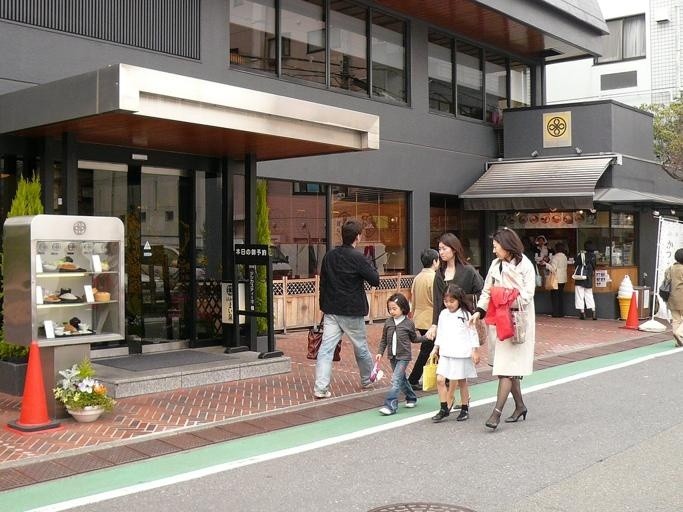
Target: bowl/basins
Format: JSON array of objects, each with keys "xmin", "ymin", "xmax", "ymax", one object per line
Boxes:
[
  {"xmin": 77, "ymin": 323, "xmax": 89, "ymax": 329},
  {"xmin": 42, "ymin": 264, "xmax": 57, "ymax": 270},
  {"xmin": 55, "ymin": 328, "xmax": 64, "ymax": 333},
  {"xmin": 93, "ymin": 293, "xmax": 111, "ymax": 302}
]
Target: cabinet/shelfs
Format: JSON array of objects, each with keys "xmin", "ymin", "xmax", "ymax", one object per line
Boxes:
[{"xmin": 2, "ymin": 215, "xmax": 126, "ymax": 349}]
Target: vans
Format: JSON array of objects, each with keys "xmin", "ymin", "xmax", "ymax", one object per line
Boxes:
[
  {"xmin": 125, "ymin": 244, "xmax": 206, "ymax": 292},
  {"xmin": 270, "ymin": 242, "xmax": 292, "ymax": 279}
]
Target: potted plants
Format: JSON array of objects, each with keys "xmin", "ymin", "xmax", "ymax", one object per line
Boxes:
[{"xmin": 254, "ymin": 179, "xmax": 277, "ymax": 353}]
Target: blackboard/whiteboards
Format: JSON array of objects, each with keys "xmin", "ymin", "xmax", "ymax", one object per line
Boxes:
[{"xmin": 656, "ymin": 217, "xmax": 683, "ymax": 295}]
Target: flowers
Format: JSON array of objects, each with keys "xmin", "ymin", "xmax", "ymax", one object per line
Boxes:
[{"xmin": 52, "ymin": 353, "xmax": 120, "ymax": 416}]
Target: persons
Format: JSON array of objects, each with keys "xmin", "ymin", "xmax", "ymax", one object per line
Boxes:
[
  {"xmin": 468, "ymin": 229, "xmax": 536, "ymax": 430},
  {"xmin": 427, "ymin": 233, "xmax": 484, "ymax": 413},
  {"xmin": 313, "ymin": 221, "xmax": 384, "ymax": 398},
  {"xmin": 430, "ymin": 284, "xmax": 480, "ymax": 422},
  {"xmin": 407, "ymin": 249, "xmax": 450, "ymax": 390},
  {"xmin": 574, "ymin": 241, "xmax": 597, "ymax": 320},
  {"xmin": 376, "ymin": 294, "xmax": 430, "ymax": 415},
  {"xmin": 535, "ymin": 235, "xmax": 552, "ymax": 265},
  {"xmin": 521, "ymin": 237, "xmax": 537, "ymax": 275},
  {"xmin": 540, "ymin": 243, "xmax": 567, "ymax": 317},
  {"xmin": 665, "ymin": 248, "xmax": 683, "ymax": 347}
]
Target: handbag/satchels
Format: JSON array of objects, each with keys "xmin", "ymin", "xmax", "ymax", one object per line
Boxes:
[
  {"xmin": 472, "ymin": 295, "xmax": 488, "ymax": 346},
  {"xmin": 570, "ymin": 251, "xmax": 588, "ymax": 281},
  {"xmin": 422, "ymin": 354, "xmax": 441, "ymax": 393},
  {"xmin": 510, "ymin": 296, "xmax": 530, "ymax": 347},
  {"xmin": 544, "ymin": 264, "xmax": 558, "ymax": 291},
  {"xmin": 658, "ymin": 277, "xmax": 674, "ymax": 302},
  {"xmin": 304, "ymin": 315, "xmax": 344, "ymax": 362}
]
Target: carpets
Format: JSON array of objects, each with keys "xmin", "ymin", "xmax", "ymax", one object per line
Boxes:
[{"xmin": 91, "ymin": 350, "xmax": 241, "ymax": 371}]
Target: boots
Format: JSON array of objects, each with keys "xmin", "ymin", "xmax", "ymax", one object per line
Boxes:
[
  {"xmin": 431, "ymin": 399, "xmax": 452, "ymax": 422},
  {"xmin": 456, "ymin": 404, "xmax": 472, "ymax": 421}
]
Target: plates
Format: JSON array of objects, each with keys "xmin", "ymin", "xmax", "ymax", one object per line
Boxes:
[
  {"xmin": 59, "ymin": 262, "xmax": 79, "ymax": 270},
  {"xmin": 44, "ymin": 296, "xmax": 60, "ymax": 302}
]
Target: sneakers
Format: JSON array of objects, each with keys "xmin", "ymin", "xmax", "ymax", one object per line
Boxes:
[
  {"xmin": 378, "ymin": 408, "xmax": 398, "ymax": 415},
  {"xmin": 410, "ymin": 382, "xmax": 423, "ymax": 391},
  {"xmin": 314, "ymin": 390, "xmax": 331, "ymax": 398},
  {"xmin": 673, "ymin": 332, "xmax": 683, "ymax": 348},
  {"xmin": 361, "ymin": 369, "xmax": 384, "ymax": 388},
  {"xmin": 592, "ymin": 313, "xmax": 598, "ymax": 321},
  {"xmin": 405, "ymin": 401, "xmax": 418, "ymax": 408},
  {"xmin": 579, "ymin": 314, "xmax": 587, "ymax": 320}
]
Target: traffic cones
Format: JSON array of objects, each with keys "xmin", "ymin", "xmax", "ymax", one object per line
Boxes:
[
  {"xmin": 619, "ymin": 291, "xmax": 641, "ymax": 333},
  {"xmin": 7, "ymin": 342, "xmax": 60, "ymax": 434}
]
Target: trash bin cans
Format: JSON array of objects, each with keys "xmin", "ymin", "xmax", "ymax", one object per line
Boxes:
[{"xmin": 634, "ymin": 286, "xmax": 651, "ymax": 319}]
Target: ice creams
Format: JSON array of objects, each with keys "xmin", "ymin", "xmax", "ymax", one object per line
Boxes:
[{"xmin": 616, "ymin": 275, "xmax": 634, "ymax": 320}]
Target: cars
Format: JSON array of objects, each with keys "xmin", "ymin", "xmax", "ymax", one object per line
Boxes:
[{"xmin": 124, "ymin": 261, "xmax": 164, "ymax": 310}]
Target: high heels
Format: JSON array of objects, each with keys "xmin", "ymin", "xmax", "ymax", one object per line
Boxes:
[
  {"xmin": 486, "ymin": 417, "xmax": 502, "ymax": 431},
  {"xmin": 505, "ymin": 408, "xmax": 529, "ymax": 424}
]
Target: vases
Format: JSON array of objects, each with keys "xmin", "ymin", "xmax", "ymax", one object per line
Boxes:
[{"xmin": 64, "ymin": 404, "xmax": 107, "ymax": 423}]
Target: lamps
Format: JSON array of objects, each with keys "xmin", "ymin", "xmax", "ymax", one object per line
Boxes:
[{"xmin": 531, "ymin": 151, "xmax": 539, "ymax": 157}]
